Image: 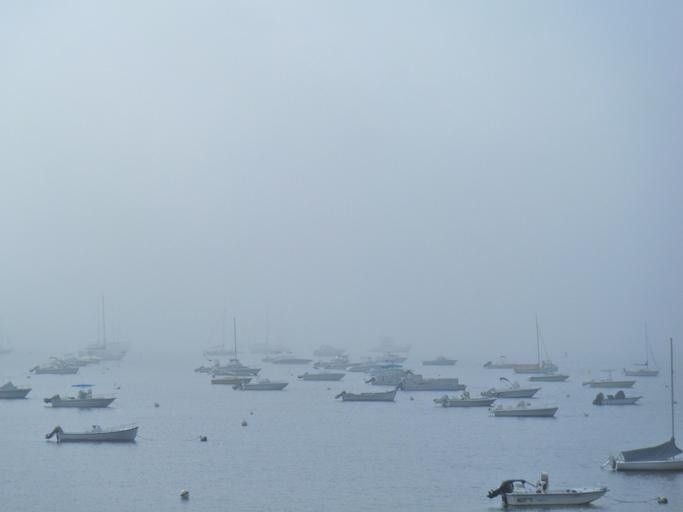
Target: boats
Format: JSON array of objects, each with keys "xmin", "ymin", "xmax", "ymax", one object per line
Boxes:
[
  {"xmin": 297, "ymin": 343, "xmax": 466, "ymax": 390},
  {"xmin": 483, "ymin": 312, "xmax": 570, "ymax": 381},
  {"xmin": 0, "ymin": 381, "xmax": 32, "ymax": 399},
  {"xmin": 335, "ymin": 387, "xmax": 399, "ymax": 402},
  {"xmin": 29, "ymin": 295, "xmax": 129, "ymax": 374},
  {"xmin": 46, "ymin": 424, "xmax": 139, "ymax": 442},
  {"xmin": 486, "ymin": 471, "xmax": 612, "ymax": 507},
  {"xmin": 194, "ymin": 316, "xmax": 290, "ymax": 390},
  {"xmin": 581, "ymin": 321, "xmax": 682, "ymax": 471},
  {"xmin": 42, "ymin": 385, "xmax": 117, "ymax": 408},
  {"xmin": 433, "ymin": 376, "xmax": 559, "ymax": 417},
  {"xmin": 261, "ymin": 351, "xmax": 313, "ymax": 364}
]
